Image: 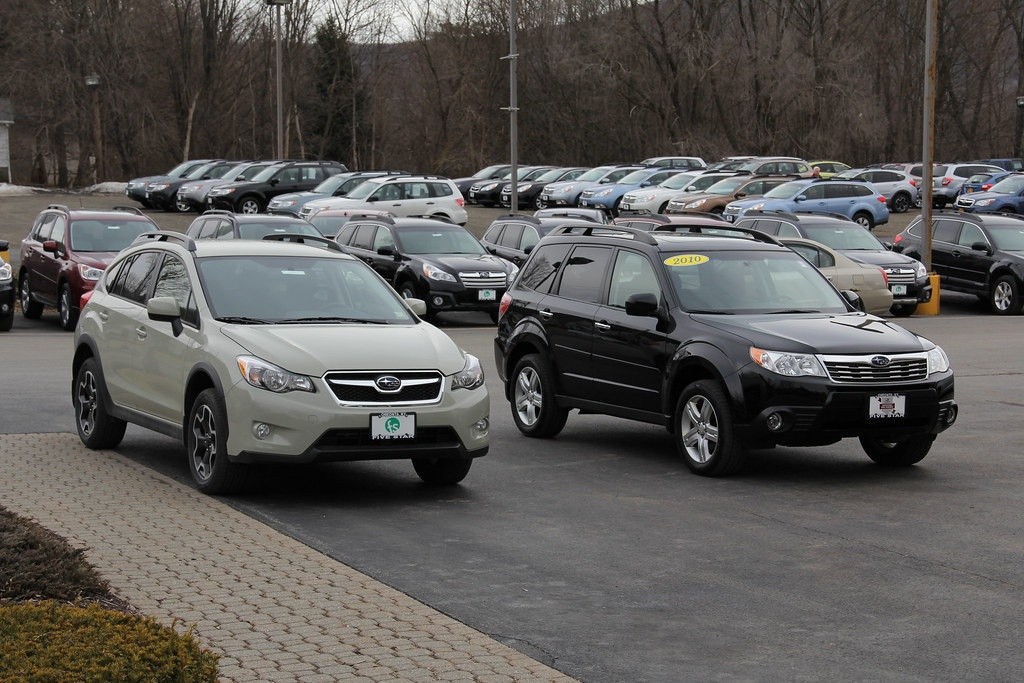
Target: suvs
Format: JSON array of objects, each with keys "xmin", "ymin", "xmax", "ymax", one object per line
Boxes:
[
  {"xmin": 71, "ymin": 230, "xmax": 489, "ymax": 499},
  {"xmin": 204, "ymin": 159, "xmax": 355, "ymax": 218},
  {"xmin": 297, "ymin": 171, "xmax": 469, "ymax": 239},
  {"xmin": 332, "ymin": 213, "xmax": 519, "ymax": 326},
  {"xmin": 125, "ymin": 160, "xmax": 201, "ymax": 209},
  {"xmin": 184, "ymin": 207, "xmax": 337, "ymax": 272},
  {"xmin": 17, "ymin": 204, "xmax": 170, "ymax": 333},
  {"xmin": 601, "ymin": 207, "xmax": 754, "ymax": 275},
  {"xmin": 477, "ymin": 211, "xmax": 608, "ymax": 272},
  {"xmin": 493, "ymin": 221, "xmax": 960, "ymax": 479},
  {"xmin": 143, "ymin": 159, "xmax": 251, "ymax": 213},
  {"xmin": 439, "ymin": 155, "xmax": 1024, "ymax": 232},
  {"xmin": 890, "ymin": 210, "xmax": 1024, "ymax": 317},
  {"xmin": 266, "ymin": 170, "xmax": 429, "ymax": 221},
  {"xmin": 177, "ymin": 158, "xmax": 316, "ymax": 220},
  {"xmin": 724, "ymin": 210, "xmax": 932, "ymax": 319}
]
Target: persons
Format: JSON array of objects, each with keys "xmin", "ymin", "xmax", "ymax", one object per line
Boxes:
[{"xmin": 811, "ymin": 166, "xmax": 822, "ymax": 178}]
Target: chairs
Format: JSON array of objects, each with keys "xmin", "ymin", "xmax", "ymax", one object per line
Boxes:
[{"xmin": 642, "ymin": 270, "xmax": 711, "ymax": 313}]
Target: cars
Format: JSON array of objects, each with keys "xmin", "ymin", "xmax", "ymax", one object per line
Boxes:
[
  {"xmin": 0, "ymin": 239, "xmax": 16, "ymax": 333},
  {"xmin": 711, "ymin": 237, "xmax": 894, "ymax": 320}
]
